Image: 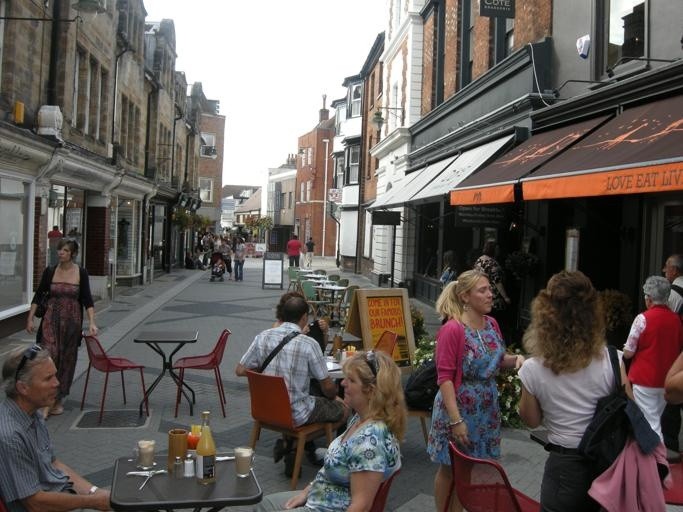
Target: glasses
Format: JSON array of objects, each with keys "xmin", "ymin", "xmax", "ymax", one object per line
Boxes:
[
  {"xmin": 364, "ymin": 349, "xmax": 377, "ymax": 377},
  {"xmin": 15, "ymin": 344, "xmax": 42, "ymax": 384},
  {"xmin": 60, "ymin": 237, "xmax": 76, "ymax": 243}
]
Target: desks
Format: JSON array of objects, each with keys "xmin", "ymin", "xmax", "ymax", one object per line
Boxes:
[{"xmin": 134, "ymin": 328, "xmax": 199, "ymax": 417}]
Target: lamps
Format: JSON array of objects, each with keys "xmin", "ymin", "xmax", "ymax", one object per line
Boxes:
[{"xmin": 371, "ymin": 106, "xmax": 403, "ymax": 131}]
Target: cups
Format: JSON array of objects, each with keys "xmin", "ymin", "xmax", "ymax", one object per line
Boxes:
[
  {"xmin": 137, "ymin": 440, "xmax": 155, "ymax": 470},
  {"xmin": 233, "ymin": 446, "xmax": 252, "ymax": 478}
]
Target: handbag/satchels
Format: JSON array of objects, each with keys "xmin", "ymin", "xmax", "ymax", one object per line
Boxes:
[
  {"xmin": 577, "ymin": 390, "xmax": 661, "ymax": 478},
  {"xmin": 405, "ymin": 360, "xmax": 440, "ymax": 411}
]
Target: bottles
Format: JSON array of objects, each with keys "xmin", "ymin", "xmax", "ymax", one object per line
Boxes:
[
  {"xmin": 173, "ymin": 456, "xmax": 183, "ymax": 480},
  {"xmin": 336, "ymin": 349, "xmax": 346, "ymax": 361},
  {"xmin": 195, "ymin": 411, "xmax": 216, "ymax": 485},
  {"xmin": 184, "ymin": 454, "xmax": 195, "ymax": 477}
]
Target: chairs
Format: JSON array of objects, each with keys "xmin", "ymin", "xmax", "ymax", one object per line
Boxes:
[
  {"xmin": 370, "ymin": 464, "xmax": 402, "ymax": 512},
  {"xmin": 659, "ymin": 463, "xmax": 683, "ymax": 505},
  {"xmin": 172, "ymin": 328, "xmax": 231, "ymax": 417},
  {"xmin": 287, "ymin": 266, "xmax": 360, "ymax": 321},
  {"xmin": 444, "ymin": 440, "xmax": 541, "ymax": 512},
  {"xmin": 404, "ymin": 409, "xmax": 432, "ymax": 447},
  {"xmin": 80, "ymin": 334, "xmax": 148, "ymax": 424},
  {"xmin": 244, "ymin": 368, "xmax": 333, "ymax": 491}
]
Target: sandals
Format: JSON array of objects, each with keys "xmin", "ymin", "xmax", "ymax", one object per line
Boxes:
[{"xmin": 274, "ymin": 438, "xmax": 302, "ymax": 478}]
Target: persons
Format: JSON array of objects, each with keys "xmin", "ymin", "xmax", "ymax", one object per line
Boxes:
[
  {"xmin": 425, "ymin": 237, "xmax": 682, "ymax": 512},
  {"xmin": 0, "ymin": 343, "xmax": 113, "ymax": 512},
  {"xmin": 197, "ymin": 224, "xmax": 248, "ymax": 282},
  {"xmin": 235, "ymin": 293, "xmax": 351, "ymax": 478},
  {"xmin": 304, "ymin": 237, "xmax": 316, "ymax": 268},
  {"xmin": 287, "ymin": 235, "xmax": 301, "ymax": 267},
  {"xmin": 27, "ymin": 237, "xmax": 97, "ymax": 415},
  {"xmin": 256, "ymin": 350, "xmax": 407, "ymax": 512},
  {"xmin": 48, "ymin": 226, "xmax": 62, "ymax": 265},
  {"xmin": 273, "ymin": 319, "xmax": 328, "ymax": 355}
]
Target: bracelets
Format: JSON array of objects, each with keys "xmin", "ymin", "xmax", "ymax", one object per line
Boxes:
[
  {"xmin": 89, "ymin": 485, "xmax": 98, "ymax": 495},
  {"xmin": 310, "ymin": 482, "xmax": 314, "ymax": 486}
]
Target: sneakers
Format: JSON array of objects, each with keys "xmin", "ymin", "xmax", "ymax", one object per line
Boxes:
[{"xmin": 50, "ymin": 398, "xmax": 65, "ymax": 414}]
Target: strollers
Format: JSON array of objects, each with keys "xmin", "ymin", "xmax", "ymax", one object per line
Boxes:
[{"xmin": 210, "ymin": 251, "xmax": 226, "ymax": 282}]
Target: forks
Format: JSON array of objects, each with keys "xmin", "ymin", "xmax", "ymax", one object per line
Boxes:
[{"xmin": 138, "ymin": 470, "xmax": 157, "ymax": 491}]
